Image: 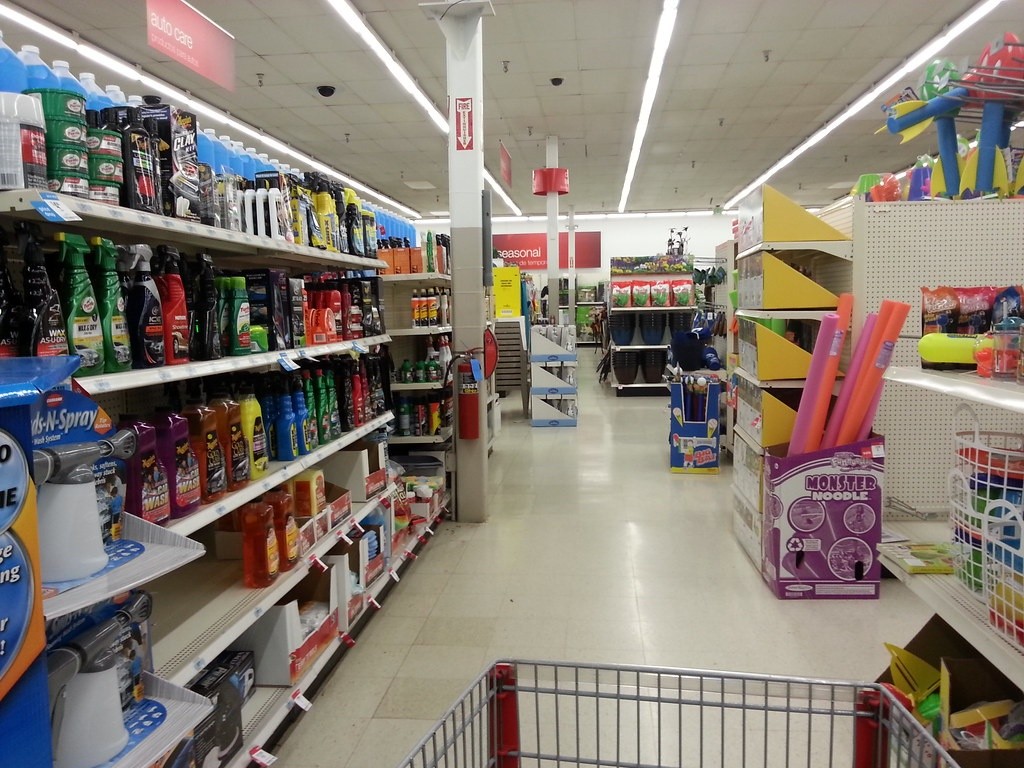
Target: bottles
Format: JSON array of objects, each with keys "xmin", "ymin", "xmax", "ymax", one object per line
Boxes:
[
  {"xmin": 362, "ymin": 508, "xmax": 390, "ymax": 571},
  {"xmin": 265, "ymin": 486, "xmax": 301, "ymax": 573},
  {"xmin": 242, "ymin": 497, "xmax": 280, "ymax": 589},
  {"xmin": 195, "ymin": 123, "xmax": 416, "ymax": 254},
  {"xmin": 182, "ymin": 400, "xmax": 226, "ymax": 505},
  {"xmin": 303, "ymin": 283, "xmax": 352, "ymax": 338},
  {"xmin": 237, "ymin": 386, "xmax": 269, "ymax": 479},
  {"xmin": 209, "ymin": 392, "xmax": 251, "ymax": 492},
  {"xmin": 146, "ymin": 405, "xmax": 201, "ymax": 518},
  {"xmin": 216, "ymin": 275, "xmax": 251, "ymax": 355},
  {"xmin": 411, "ymin": 286, "xmax": 448, "ymax": 325},
  {"xmin": 115, "ymin": 412, "xmax": 168, "ymax": 525},
  {"xmin": 0, "ymin": 30, "xmax": 165, "ymax": 215}
]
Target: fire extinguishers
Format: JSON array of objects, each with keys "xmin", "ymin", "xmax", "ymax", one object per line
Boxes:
[{"xmin": 440, "ymin": 341, "xmax": 483, "ymax": 441}]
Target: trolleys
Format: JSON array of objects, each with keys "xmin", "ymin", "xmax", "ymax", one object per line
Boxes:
[{"xmin": 394, "ymin": 659, "xmax": 963, "ymax": 768}]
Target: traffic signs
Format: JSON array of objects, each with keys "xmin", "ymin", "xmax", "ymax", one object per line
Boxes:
[{"xmin": 454, "ymin": 98, "xmax": 473, "ymax": 151}]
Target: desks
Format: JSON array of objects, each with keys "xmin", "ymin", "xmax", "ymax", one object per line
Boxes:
[{"xmin": 560, "ymin": 255, "xmax": 698, "ymax": 398}]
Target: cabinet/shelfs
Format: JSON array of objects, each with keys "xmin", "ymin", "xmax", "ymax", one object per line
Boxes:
[
  {"xmin": 0, "ymin": 7, "xmax": 449, "ymax": 768},
  {"xmin": 876, "ymin": 63, "xmax": 1024, "ymax": 703},
  {"xmin": 696, "ymin": 241, "xmax": 738, "ymax": 454},
  {"xmin": 377, "ymin": 271, "xmax": 457, "ymax": 518},
  {"xmin": 735, "ymin": 198, "xmax": 1023, "ymax": 580}
]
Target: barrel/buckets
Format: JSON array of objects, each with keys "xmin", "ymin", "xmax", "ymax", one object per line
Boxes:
[
  {"xmin": 610, "ymin": 313, "xmax": 691, "ymax": 345},
  {"xmin": 611, "ymin": 349, "xmax": 668, "ymax": 384}
]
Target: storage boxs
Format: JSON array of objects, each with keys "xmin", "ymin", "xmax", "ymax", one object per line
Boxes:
[
  {"xmin": 668, "ymin": 183, "xmax": 1023, "ymax": 768},
  {"xmin": 529, "ymin": 328, "xmax": 578, "ymax": 428},
  {"xmin": 209, "ymin": 440, "xmax": 443, "ymax": 688},
  {"xmin": 492, "ymin": 266, "xmax": 522, "ymax": 317}
]
